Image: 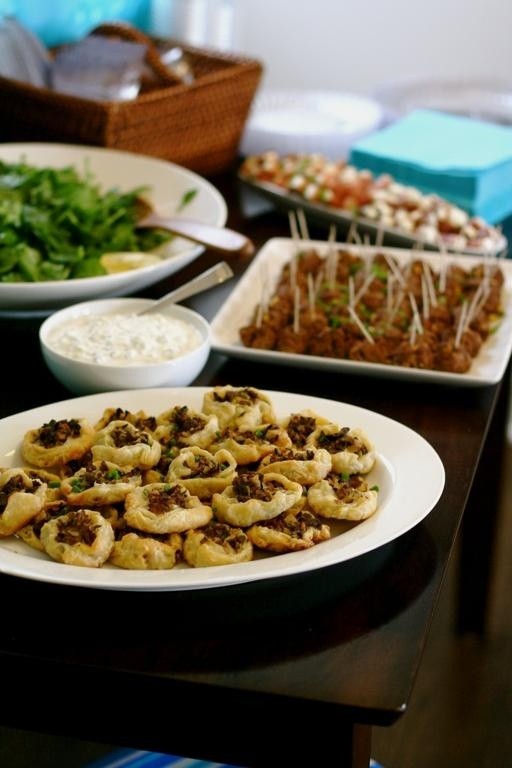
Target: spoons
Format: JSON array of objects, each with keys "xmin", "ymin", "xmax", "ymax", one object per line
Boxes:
[{"xmin": 131, "ymin": 198, "xmax": 256, "ymax": 259}]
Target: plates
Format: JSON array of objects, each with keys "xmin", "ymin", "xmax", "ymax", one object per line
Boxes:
[
  {"xmin": 236, "ymin": 151, "xmax": 507, "ymax": 257},
  {"xmin": 236, "ymin": 93, "xmax": 385, "ymax": 161},
  {"xmin": 0, "ymin": 385, "xmax": 448, "ymax": 594},
  {"xmin": 208, "ymin": 236, "xmax": 512, "ymax": 388},
  {"xmin": 0, "ymin": 140, "xmax": 228, "ymax": 319}
]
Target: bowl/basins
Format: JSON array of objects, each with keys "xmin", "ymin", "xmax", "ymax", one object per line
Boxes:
[{"xmin": 38, "ymin": 297, "xmax": 214, "ymax": 395}]
